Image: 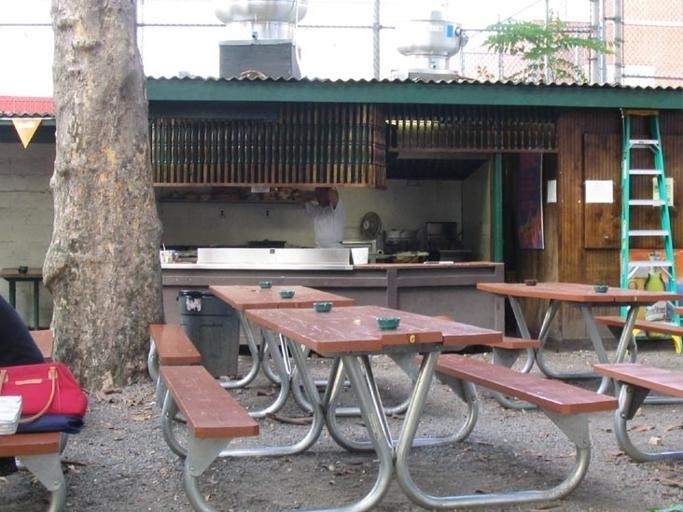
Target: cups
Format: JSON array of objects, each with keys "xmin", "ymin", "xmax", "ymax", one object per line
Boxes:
[
  {"xmin": 351, "ymin": 247, "xmax": 369, "ymax": 265},
  {"xmin": 159, "ymin": 250, "xmax": 178, "ymax": 264}
]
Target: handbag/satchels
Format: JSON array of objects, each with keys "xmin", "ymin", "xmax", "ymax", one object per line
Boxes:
[{"xmin": 0, "ymin": 362, "xmax": 87, "ymax": 424}]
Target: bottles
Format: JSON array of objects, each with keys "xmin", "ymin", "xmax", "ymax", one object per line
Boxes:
[{"xmin": 644, "ymin": 251, "xmax": 667, "ymax": 337}]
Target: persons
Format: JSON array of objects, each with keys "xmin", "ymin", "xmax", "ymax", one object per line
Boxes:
[{"xmin": 1, "ymin": 297, "xmax": 47, "ymax": 484}]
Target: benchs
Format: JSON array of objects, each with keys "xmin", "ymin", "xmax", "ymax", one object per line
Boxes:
[
  {"xmin": 0, "ymin": 432, "xmax": 68, "ymax": 512},
  {"xmin": 26, "ymin": 330, "xmax": 54, "ymax": 359}
]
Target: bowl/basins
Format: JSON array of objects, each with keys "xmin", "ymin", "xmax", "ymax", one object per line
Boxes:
[
  {"xmin": 279, "ymin": 291, "xmax": 295, "ymax": 298},
  {"xmin": 593, "ymin": 285, "xmax": 609, "ymax": 293},
  {"xmin": 259, "ymin": 281, "xmax": 272, "ymax": 288},
  {"xmin": 313, "ymin": 301, "xmax": 333, "ymax": 313},
  {"xmin": 525, "ymin": 279, "xmax": 537, "ymax": 287},
  {"xmin": 376, "ymin": 316, "xmax": 400, "ymax": 330}
]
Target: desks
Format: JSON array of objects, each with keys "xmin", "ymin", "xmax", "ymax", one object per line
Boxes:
[
  {"xmin": 476, "ymin": 282, "xmax": 683, "ymax": 410},
  {"xmin": 207, "ymin": 284, "xmax": 355, "ymax": 417},
  {"xmin": 0, "ymin": 268, "xmax": 42, "ymax": 330}
]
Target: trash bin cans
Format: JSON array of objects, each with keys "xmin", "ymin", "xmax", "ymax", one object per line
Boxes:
[{"xmin": 176, "ymin": 289, "xmax": 240, "ymax": 379}]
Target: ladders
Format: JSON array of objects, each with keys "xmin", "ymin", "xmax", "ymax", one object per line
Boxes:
[{"xmin": 621, "ymin": 108, "xmax": 681, "ymax": 362}]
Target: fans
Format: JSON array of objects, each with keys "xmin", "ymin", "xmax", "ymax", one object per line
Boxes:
[{"xmin": 361, "ymin": 212, "xmax": 382, "ymax": 239}]
